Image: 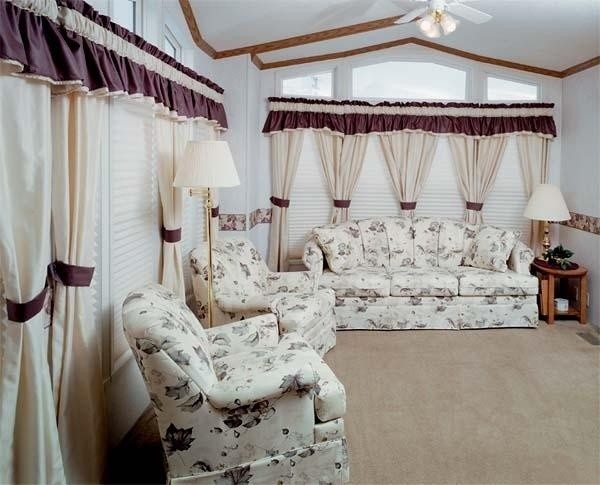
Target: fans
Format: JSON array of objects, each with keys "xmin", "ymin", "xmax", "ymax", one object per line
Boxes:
[{"xmin": 393, "ymin": 0, "xmax": 493, "ymax": 26}]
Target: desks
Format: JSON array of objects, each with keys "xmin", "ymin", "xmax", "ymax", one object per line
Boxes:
[{"xmin": 532, "ymin": 257, "xmax": 589, "ymax": 326}]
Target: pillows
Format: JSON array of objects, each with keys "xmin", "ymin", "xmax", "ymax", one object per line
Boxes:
[
  {"xmin": 313, "ymin": 218, "xmax": 367, "ymax": 274},
  {"xmin": 461, "ymin": 219, "xmax": 522, "ymax": 272}
]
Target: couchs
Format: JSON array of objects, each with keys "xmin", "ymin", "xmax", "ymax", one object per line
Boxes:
[
  {"xmin": 119, "ymin": 282, "xmax": 352, "ymax": 485},
  {"xmin": 187, "ymin": 236, "xmax": 337, "ymax": 362},
  {"xmin": 297, "ymin": 215, "xmax": 540, "ymax": 332}
]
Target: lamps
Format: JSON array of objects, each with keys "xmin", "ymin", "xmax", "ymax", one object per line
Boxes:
[
  {"xmin": 523, "ymin": 182, "xmax": 571, "ymax": 260},
  {"xmin": 419, "ymin": 8, "xmax": 458, "ymax": 38},
  {"xmin": 174, "ymin": 135, "xmax": 242, "ymax": 330}
]
